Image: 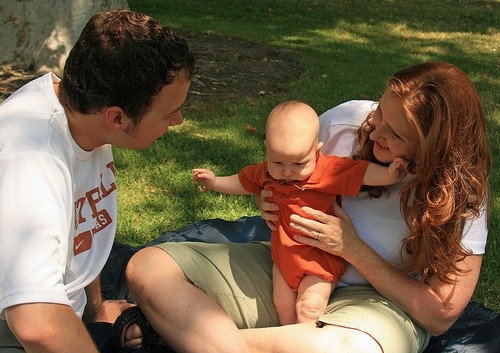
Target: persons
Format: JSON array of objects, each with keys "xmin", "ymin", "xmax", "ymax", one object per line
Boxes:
[
  {"xmin": 191, "ymin": 99, "xmax": 411, "ymax": 327},
  {"xmin": 125, "ymin": 62, "xmax": 490, "ymax": 353},
  {"xmin": 0, "ymin": 8, "xmax": 196, "ymax": 353}
]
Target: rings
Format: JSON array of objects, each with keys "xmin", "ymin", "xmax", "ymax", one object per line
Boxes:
[{"xmin": 314, "ymin": 231, "xmax": 320, "ymax": 240}]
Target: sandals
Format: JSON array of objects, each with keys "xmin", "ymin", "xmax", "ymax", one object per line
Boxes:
[{"xmin": 109, "ymin": 305, "xmax": 167, "ymax": 353}]
